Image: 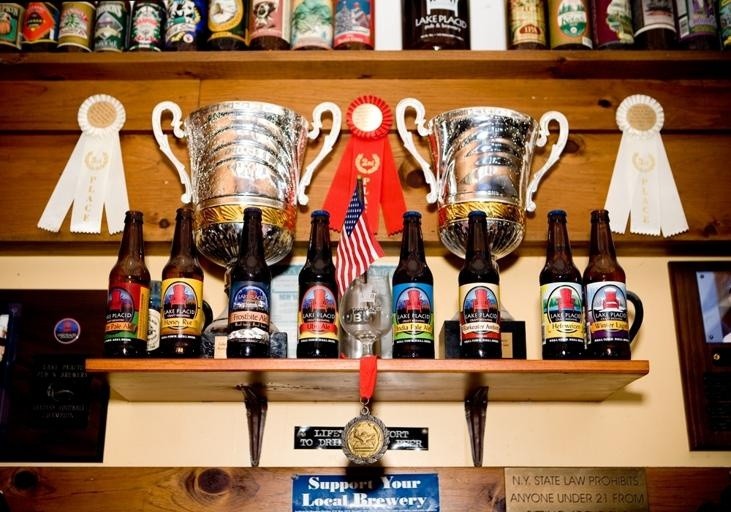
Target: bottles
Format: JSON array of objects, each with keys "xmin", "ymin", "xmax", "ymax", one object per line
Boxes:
[
  {"xmin": 393, "ymin": 211, "xmax": 437, "ymax": 358},
  {"xmin": 539, "ymin": 210, "xmax": 587, "ymax": 360},
  {"xmin": 105, "ymin": 210, "xmax": 150, "ymax": 359},
  {"xmin": 159, "ymin": 207, "xmax": 205, "ymax": 360},
  {"xmin": 227, "ymin": 207, "xmax": 271, "ymax": 358},
  {"xmin": 583, "ymin": 208, "xmax": 632, "ymax": 360},
  {"xmin": 298, "ymin": 210, "xmax": 341, "ymax": 358},
  {"xmin": 144, "ymin": 280, "xmax": 161, "ymax": 358},
  {"xmin": 0, "ymin": 0, "xmax": 730, "ymax": 57},
  {"xmin": 459, "ymin": 210, "xmax": 503, "ymax": 361}
]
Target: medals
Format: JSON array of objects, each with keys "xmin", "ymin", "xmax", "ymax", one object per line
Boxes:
[{"xmin": 340, "ymin": 413, "xmax": 390, "ymax": 465}]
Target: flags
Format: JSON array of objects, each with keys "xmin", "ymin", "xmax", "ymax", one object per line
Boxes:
[{"xmin": 335, "ymin": 180, "xmax": 387, "ymax": 299}]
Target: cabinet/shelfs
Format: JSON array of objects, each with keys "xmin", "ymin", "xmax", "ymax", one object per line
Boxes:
[{"xmin": 0, "ymin": 45, "xmax": 730, "ymax": 466}]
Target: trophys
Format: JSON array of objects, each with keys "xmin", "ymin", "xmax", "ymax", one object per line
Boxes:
[
  {"xmin": 151, "ymin": 101, "xmax": 343, "ymax": 358},
  {"xmin": 394, "ymin": 98, "xmax": 569, "ymax": 358}
]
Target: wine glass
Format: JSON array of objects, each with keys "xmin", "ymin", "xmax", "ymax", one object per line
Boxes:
[{"xmin": 340, "ymin": 278, "xmax": 391, "ymax": 358}]
